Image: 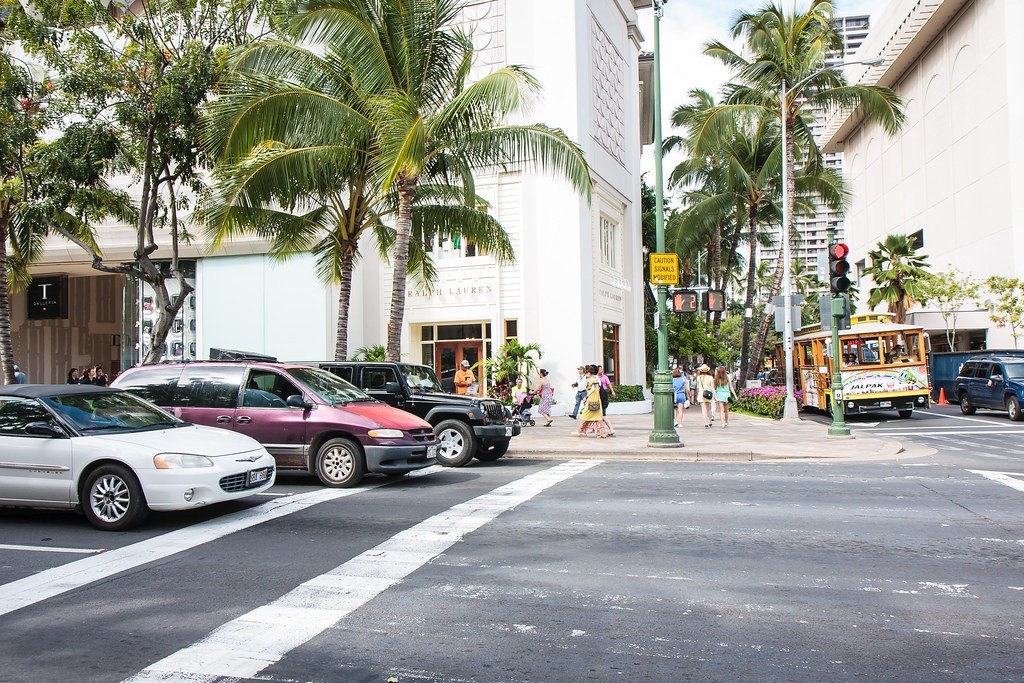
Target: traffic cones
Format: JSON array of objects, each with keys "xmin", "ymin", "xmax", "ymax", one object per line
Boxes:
[{"xmin": 936, "ymin": 388, "xmax": 949, "ymax": 405}]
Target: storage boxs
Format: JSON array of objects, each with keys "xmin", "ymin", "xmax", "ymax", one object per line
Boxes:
[{"xmin": 745, "ymin": 379, "xmax": 761, "ymax": 389}]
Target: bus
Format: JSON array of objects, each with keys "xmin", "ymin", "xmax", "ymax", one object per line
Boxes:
[{"xmin": 774, "ymin": 312, "xmax": 931, "ymax": 420}]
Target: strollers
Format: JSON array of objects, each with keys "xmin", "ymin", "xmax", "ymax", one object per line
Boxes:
[{"xmin": 511, "ymin": 393, "xmax": 536, "ymax": 428}]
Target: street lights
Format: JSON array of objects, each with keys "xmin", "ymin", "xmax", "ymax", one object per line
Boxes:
[{"xmin": 781, "ymin": 56, "xmax": 886, "ymax": 409}]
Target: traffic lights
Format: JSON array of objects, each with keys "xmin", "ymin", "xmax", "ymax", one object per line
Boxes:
[
  {"xmin": 832, "ymin": 296, "xmax": 847, "ymax": 319},
  {"xmin": 671, "ymin": 290, "xmax": 699, "ymax": 313},
  {"xmin": 702, "ymin": 290, "xmax": 728, "ymax": 313},
  {"xmin": 828, "ymin": 244, "xmax": 852, "ymax": 294}
]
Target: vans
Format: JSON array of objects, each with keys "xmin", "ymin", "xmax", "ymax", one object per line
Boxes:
[{"xmin": 107, "ymin": 355, "xmax": 447, "ymax": 488}]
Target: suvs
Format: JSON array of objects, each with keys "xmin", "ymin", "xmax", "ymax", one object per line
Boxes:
[
  {"xmin": 263, "ymin": 356, "xmax": 525, "ymax": 467},
  {"xmin": 953, "ymin": 356, "xmax": 1024, "ymax": 421}
]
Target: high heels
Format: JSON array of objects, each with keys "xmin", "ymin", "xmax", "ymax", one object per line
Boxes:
[{"xmin": 704, "ymin": 422, "xmax": 713, "ymax": 429}]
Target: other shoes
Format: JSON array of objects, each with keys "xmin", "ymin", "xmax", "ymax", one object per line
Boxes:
[
  {"xmin": 721, "ymin": 422, "xmax": 729, "ymax": 429},
  {"xmin": 674, "ymin": 421, "xmax": 684, "ymax": 428},
  {"xmin": 577, "ymin": 432, "xmax": 587, "ymax": 437},
  {"xmin": 544, "ymin": 419, "xmax": 553, "ymax": 426},
  {"xmin": 597, "ymin": 433, "xmax": 610, "ymax": 438},
  {"xmin": 607, "ymin": 430, "xmax": 616, "ymax": 437},
  {"xmin": 569, "ymin": 415, "xmax": 576, "ymax": 419}
]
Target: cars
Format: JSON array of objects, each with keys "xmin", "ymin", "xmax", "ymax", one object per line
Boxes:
[{"xmin": 0, "ymin": 381, "xmax": 278, "ymax": 533}]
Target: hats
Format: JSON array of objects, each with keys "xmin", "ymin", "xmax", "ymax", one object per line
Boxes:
[
  {"xmin": 577, "ymin": 366, "xmax": 584, "ymax": 370},
  {"xmin": 461, "ymin": 359, "xmax": 470, "ymax": 366},
  {"xmin": 14, "ymin": 365, "xmax": 20, "ymax": 371},
  {"xmin": 699, "ymin": 364, "xmax": 711, "ymax": 372}
]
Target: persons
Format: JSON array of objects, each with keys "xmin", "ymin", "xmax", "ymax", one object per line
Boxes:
[
  {"xmin": 67, "ymin": 365, "xmax": 123, "ymax": 387},
  {"xmin": 884, "ymin": 345, "xmax": 902, "ymax": 363},
  {"xmin": 175, "ymin": 347, "xmax": 182, "ymax": 356},
  {"xmin": 453, "ymin": 358, "xmax": 477, "ymax": 396},
  {"xmin": 379, "ymin": 371, "xmax": 394, "ymax": 389},
  {"xmin": 842, "ymin": 353, "xmax": 858, "ymax": 366},
  {"xmin": 416, "ymin": 372, "xmax": 432, "ymax": 388},
  {"xmin": 145, "ymin": 303, "xmax": 153, "ymax": 310},
  {"xmin": 511, "ymin": 369, "xmax": 553, "ymax": 427},
  {"xmin": 569, "ymin": 365, "xmax": 616, "ymax": 438},
  {"xmin": 13, "ymin": 365, "xmax": 26, "ymax": 384},
  {"xmin": 750, "ymin": 367, "xmax": 770, "ymax": 383},
  {"xmin": 674, "ymin": 364, "xmax": 739, "ymax": 429},
  {"xmin": 988, "ymin": 367, "xmax": 1016, "ymax": 386}
]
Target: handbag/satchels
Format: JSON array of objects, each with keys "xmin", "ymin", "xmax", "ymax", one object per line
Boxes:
[
  {"xmin": 728, "ymin": 397, "xmax": 734, "ymax": 403},
  {"xmin": 588, "ymin": 402, "xmax": 600, "ymax": 411},
  {"xmin": 703, "ymin": 390, "xmax": 713, "ymax": 400},
  {"xmin": 684, "ymin": 399, "xmax": 691, "ymax": 409}
]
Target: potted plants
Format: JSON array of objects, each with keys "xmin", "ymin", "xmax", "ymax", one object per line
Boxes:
[
  {"xmin": 471, "ymin": 338, "xmax": 565, "ymax": 417},
  {"xmin": 605, "ymin": 384, "xmax": 652, "ymax": 414}
]
